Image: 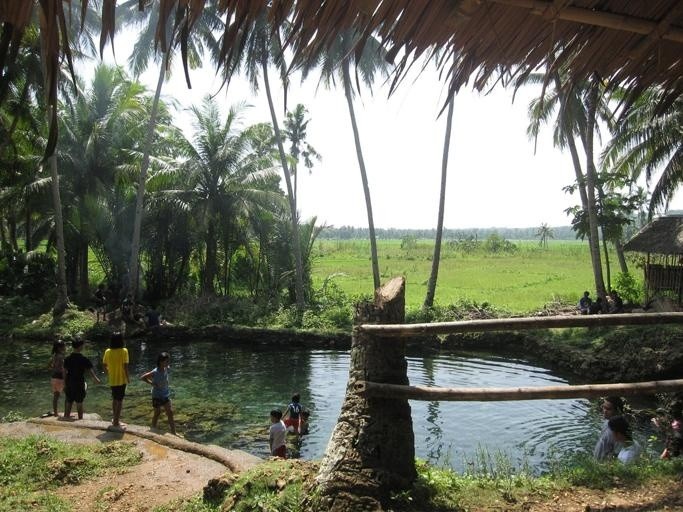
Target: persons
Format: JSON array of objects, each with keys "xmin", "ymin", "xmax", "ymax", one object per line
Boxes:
[
  {"xmin": 268, "ymin": 409, "xmax": 287, "ymax": 459},
  {"xmin": 279, "ymin": 393, "xmax": 303, "ymax": 433},
  {"xmin": 137, "ymin": 352, "xmax": 183, "ymax": 439},
  {"xmin": 121, "ymin": 293, "xmax": 134, "ymax": 324},
  {"xmin": 593, "ymin": 397, "xmax": 624, "ymax": 464},
  {"xmin": 46, "ymin": 339, "xmax": 75, "ymax": 417},
  {"xmin": 650, "ymin": 403, "xmax": 682, "ymax": 459},
  {"xmin": 579, "ymin": 291, "xmax": 591, "ymax": 314},
  {"xmin": 102, "ymin": 331, "xmax": 131, "ymax": 426},
  {"xmin": 606, "ymin": 416, "xmax": 646, "ymax": 467},
  {"xmin": 588, "ymin": 297, "xmax": 602, "ymax": 314},
  {"xmin": 604, "ymin": 289, "xmax": 622, "ymax": 313},
  {"xmin": 62, "ymin": 336, "xmax": 100, "ymax": 420},
  {"xmin": 142, "ymin": 304, "xmax": 161, "ymax": 329},
  {"xmin": 298, "ymin": 409, "xmax": 309, "ymax": 434},
  {"xmin": 93, "ymin": 283, "xmax": 108, "ymax": 324}
]
